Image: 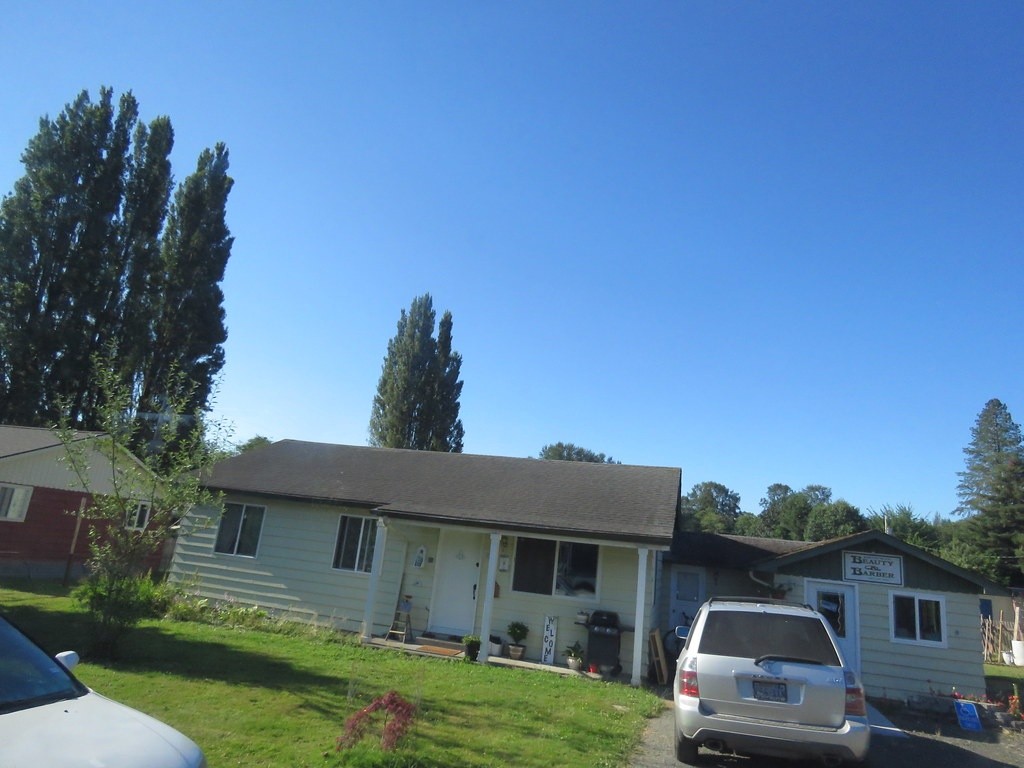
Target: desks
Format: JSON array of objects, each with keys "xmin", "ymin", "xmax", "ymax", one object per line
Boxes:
[{"xmin": 984, "ymin": 662, "xmax": 1024, "ymax": 697}]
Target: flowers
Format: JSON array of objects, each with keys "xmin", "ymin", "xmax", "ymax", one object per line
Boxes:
[
  {"xmin": 1007, "ymin": 696, "xmax": 1024, "ymax": 721},
  {"xmin": 925, "ymin": 679, "xmax": 1001, "ymax": 704}
]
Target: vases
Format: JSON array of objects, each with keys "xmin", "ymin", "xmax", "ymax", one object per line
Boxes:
[
  {"xmin": 1011, "ymin": 641, "xmax": 1024, "ymax": 667},
  {"xmin": 589, "ymin": 665, "xmax": 597, "ymax": 672},
  {"xmin": 1011, "ymin": 721, "xmax": 1024, "ymax": 731},
  {"xmin": 575, "ymin": 612, "xmax": 588, "ymax": 624},
  {"xmin": 599, "ymin": 665, "xmax": 614, "ymax": 674},
  {"xmin": 924, "ymin": 695, "xmax": 1006, "ymax": 721},
  {"xmin": 996, "ymin": 712, "xmax": 1012, "ymax": 723}
]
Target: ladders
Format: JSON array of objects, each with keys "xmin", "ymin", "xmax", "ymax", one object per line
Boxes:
[{"xmin": 384, "ymin": 595, "xmax": 414, "ymax": 644}]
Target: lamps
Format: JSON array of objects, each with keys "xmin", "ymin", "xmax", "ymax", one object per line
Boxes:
[{"xmin": 502, "ymin": 536, "xmax": 508, "ymax": 546}]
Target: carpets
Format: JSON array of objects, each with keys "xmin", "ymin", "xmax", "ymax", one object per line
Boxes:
[{"xmin": 417, "ymin": 644, "xmax": 463, "ymax": 657}]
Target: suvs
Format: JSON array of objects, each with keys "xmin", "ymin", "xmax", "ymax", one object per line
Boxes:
[{"xmin": 673, "ymin": 597, "xmax": 872, "ymax": 768}]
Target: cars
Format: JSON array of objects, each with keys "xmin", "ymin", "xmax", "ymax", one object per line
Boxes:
[{"xmin": 0, "ymin": 614, "xmax": 210, "ymax": 768}]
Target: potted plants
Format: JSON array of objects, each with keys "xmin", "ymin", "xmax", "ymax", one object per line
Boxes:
[
  {"xmin": 506, "ymin": 621, "xmax": 529, "ymax": 660},
  {"xmin": 462, "ymin": 635, "xmax": 481, "ymax": 661},
  {"xmin": 1000, "ymin": 650, "xmax": 1013, "ymax": 666},
  {"xmin": 561, "ymin": 640, "xmax": 585, "ymax": 670},
  {"xmin": 488, "ymin": 634, "xmax": 504, "ymax": 656}
]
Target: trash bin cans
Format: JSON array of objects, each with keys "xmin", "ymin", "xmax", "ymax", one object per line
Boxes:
[{"xmin": 588, "ymin": 610, "xmax": 621, "ymax": 676}]
